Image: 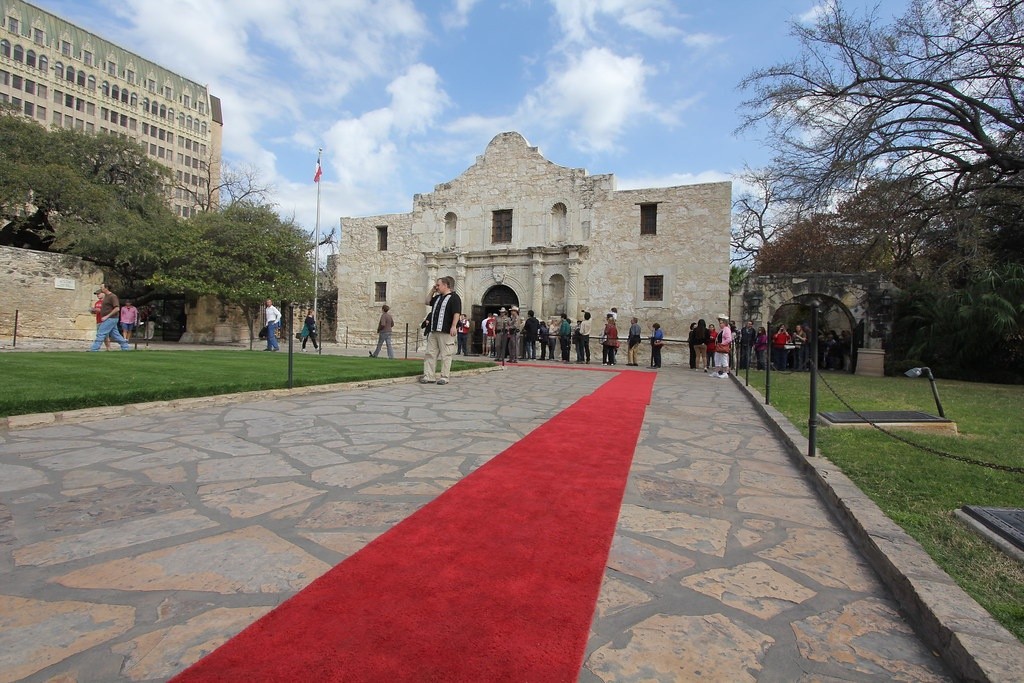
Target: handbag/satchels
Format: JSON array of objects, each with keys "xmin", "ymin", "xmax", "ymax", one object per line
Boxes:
[
  {"xmin": 784, "ymin": 341, "xmax": 795, "ymax": 350},
  {"xmin": 795, "ymin": 340, "xmax": 802, "ymax": 348},
  {"xmin": 259, "ymin": 327, "xmax": 268, "ymax": 339},
  {"xmin": 716, "ymin": 343, "xmax": 730, "ymax": 353}
]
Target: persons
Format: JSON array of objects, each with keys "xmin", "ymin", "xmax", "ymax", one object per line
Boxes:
[
  {"xmin": 579, "ymin": 310, "xmax": 592, "ymax": 364},
  {"xmin": 263, "ymin": 299, "xmax": 281, "ymax": 352},
  {"xmin": 602, "ymin": 308, "xmax": 620, "ymax": 365},
  {"xmin": 481, "ymin": 306, "xmax": 572, "ymax": 363},
  {"xmin": 119, "ymin": 299, "xmax": 138, "ymax": 342},
  {"xmin": 301, "ymin": 310, "xmax": 322, "ymax": 352},
  {"xmin": 420, "ymin": 277, "xmax": 461, "ymax": 385},
  {"xmin": 651, "ymin": 323, "xmax": 663, "ymax": 368},
  {"xmin": 88, "ymin": 289, "xmax": 111, "ymax": 351},
  {"xmin": 625, "ymin": 317, "xmax": 641, "ymax": 366},
  {"xmin": 455, "ymin": 314, "xmax": 470, "ymax": 356},
  {"xmin": 143, "ymin": 305, "xmax": 157, "ymax": 341},
  {"xmin": 689, "ymin": 313, "xmax": 851, "ymax": 379},
  {"xmin": 369, "ymin": 305, "xmax": 395, "ymax": 358},
  {"xmin": 574, "ymin": 320, "xmax": 583, "ymax": 363},
  {"xmin": 86, "ymin": 282, "xmax": 129, "ymax": 352}
]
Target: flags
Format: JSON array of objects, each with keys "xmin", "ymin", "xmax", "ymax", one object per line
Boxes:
[{"xmin": 314, "ymin": 157, "xmax": 322, "ymax": 183}]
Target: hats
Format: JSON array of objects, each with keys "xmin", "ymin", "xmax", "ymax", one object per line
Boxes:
[
  {"xmin": 717, "ymin": 313, "xmax": 730, "ymax": 319},
  {"xmin": 498, "ymin": 307, "xmax": 508, "ymax": 313},
  {"xmin": 509, "ymin": 306, "xmax": 519, "ymax": 311},
  {"xmin": 94, "ymin": 289, "xmax": 103, "ymax": 294}
]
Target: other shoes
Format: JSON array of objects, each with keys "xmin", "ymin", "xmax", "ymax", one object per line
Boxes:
[
  {"xmin": 437, "ymin": 378, "xmax": 449, "ymax": 385},
  {"xmin": 265, "ymin": 346, "xmax": 280, "ymax": 352},
  {"xmin": 456, "ymin": 352, "xmax": 460, "ymax": 354},
  {"xmin": 719, "ymin": 372, "xmax": 729, "ymax": 379},
  {"xmin": 316, "ymin": 348, "xmax": 322, "ymax": 352},
  {"xmin": 515, "ymin": 360, "xmax": 518, "ymax": 364},
  {"xmin": 709, "ymin": 371, "xmax": 719, "ymax": 377},
  {"xmin": 301, "ymin": 349, "xmax": 308, "ymax": 352},
  {"xmin": 506, "ymin": 359, "xmax": 513, "ymax": 362},
  {"xmin": 420, "ymin": 377, "xmax": 435, "ymax": 383},
  {"xmin": 704, "ymin": 368, "xmax": 708, "ymax": 372},
  {"xmin": 494, "ymin": 358, "xmax": 500, "ymax": 361},
  {"xmin": 463, "ymin": 354, "xmax": 468, "ymax": 356},
  {"xmin": 518, "ymin": 354, "xmax": 661, "ymax": 369}
]
